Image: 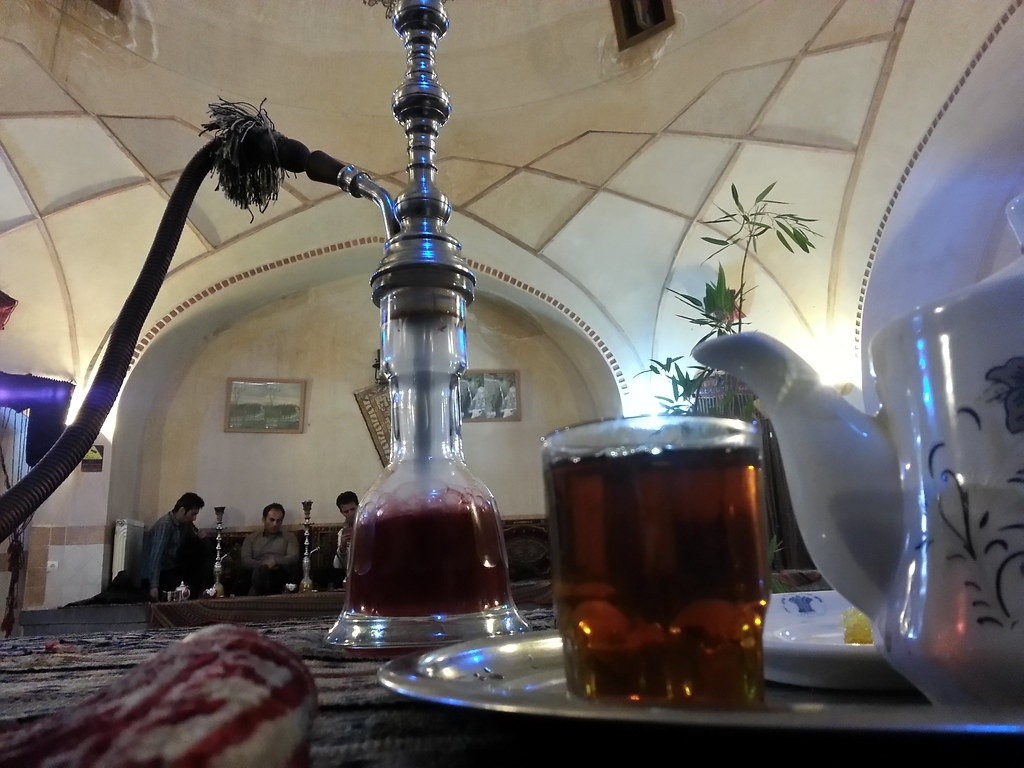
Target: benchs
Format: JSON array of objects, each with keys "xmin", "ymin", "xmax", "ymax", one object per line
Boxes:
[{"xmin": 143, "ymin": 517, "xmax": 554, "ymax": 596}]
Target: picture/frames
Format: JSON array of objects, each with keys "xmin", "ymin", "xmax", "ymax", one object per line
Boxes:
[
  {"xmin": 222, "ymin": 376, "xmax": 307, "ymax": 434},
  {"xmin": 459, "ymin": 369, "xmax": 523, "ymax": 423}
]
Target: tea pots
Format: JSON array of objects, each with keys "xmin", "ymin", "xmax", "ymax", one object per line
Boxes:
[
  {"xmin": 689, "ymin": 188, "xmax": 1024, "ymax": 711},
  {"xmin": 175, "ymin": 581, "xmax": 190, "ymax": 601}
]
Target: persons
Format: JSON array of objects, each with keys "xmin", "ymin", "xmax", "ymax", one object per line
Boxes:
[
  {"xmin": 319, "ymin": 490, "xmax": 359, "ymax": 592},
  {"xmin": 239, "ymin": 503, "xmax": 300, "ymax": 597},
  {"xmin": 129, "ymin": 492, "xmax": 208, "ymax": 604}
]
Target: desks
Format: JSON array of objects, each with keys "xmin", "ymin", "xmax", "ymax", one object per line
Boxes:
[
  {"xmin": 148, "ymin": 569, "xmax": 834, "ymax": 630},
  {"xmin": 0, "ymin": 611, "xmax": 1024, "ymax": 768}
]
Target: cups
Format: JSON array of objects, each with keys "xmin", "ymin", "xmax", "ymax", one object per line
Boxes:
[
  {"xmin": 539, "ymin": 412, "xmax": 770, "ymax": 712},
  {"xmin": 167, "ymin": 591, "xmax": 182, "ymax": 602}
]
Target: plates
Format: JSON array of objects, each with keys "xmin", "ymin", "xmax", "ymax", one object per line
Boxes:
[
  {"xmin": 763, "ymin": 590, "xmax": 915, "ymax": 690},
  {"xmin": 376, "ymin": 630, "xmax": 1024, "ymax": 734}
]
